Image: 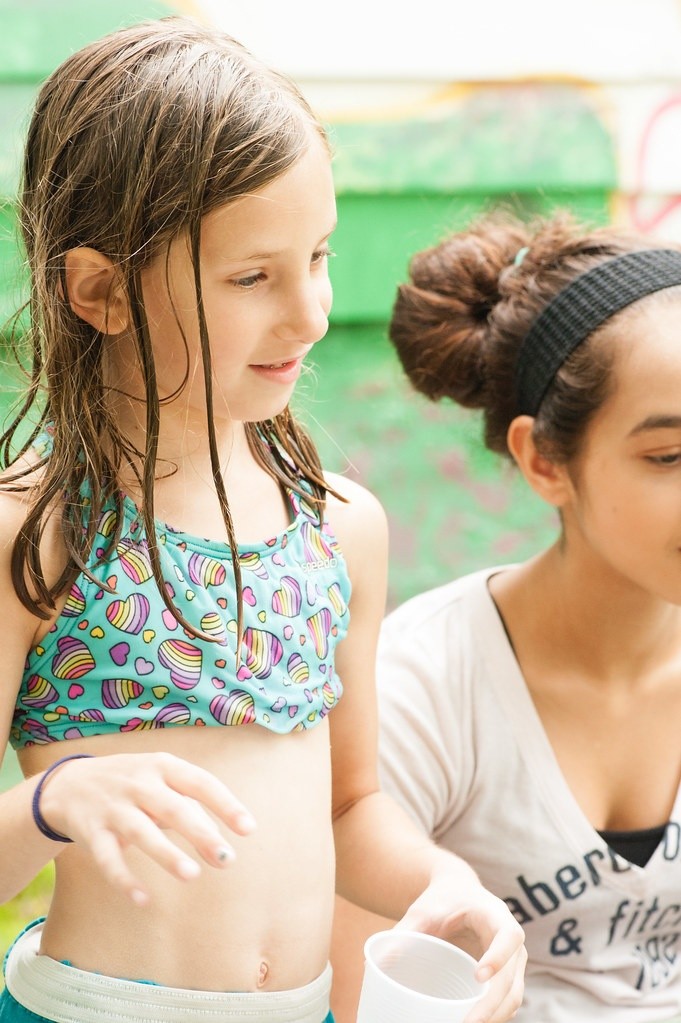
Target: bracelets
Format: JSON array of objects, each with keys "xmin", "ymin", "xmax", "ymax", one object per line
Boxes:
[{"xmin": 31, "ymin": 752, "xmax": 98, "ymax": 845}]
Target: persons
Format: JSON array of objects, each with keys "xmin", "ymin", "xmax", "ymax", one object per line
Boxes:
[
  {"xmin": 0, "ymin": 17, "xmax": 529, "ymax": 1023},
  {"xmin": 328, "ymin": 190, "xmax": 681, "ymax": 1023}
]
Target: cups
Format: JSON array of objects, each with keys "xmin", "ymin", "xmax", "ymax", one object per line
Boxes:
[{"xmin": 356, "ymin": 929, "xmax": 491, "ymax": 1023}]
